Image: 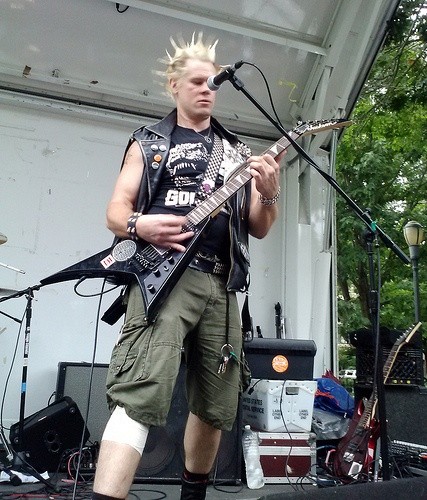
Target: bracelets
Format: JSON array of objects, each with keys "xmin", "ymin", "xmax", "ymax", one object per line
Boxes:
[
  {"xmin": 127, "ymin": 212, "xmax": 143, "ymax": 241},
  {"xmin": 258, "ymin": 186, "xmax": 281, "ymax": 206}
]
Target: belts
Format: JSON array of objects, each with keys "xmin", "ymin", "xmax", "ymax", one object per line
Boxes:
[{"xmin": 188, "ymin": 255, "xmax": 228, "ymax": 275}]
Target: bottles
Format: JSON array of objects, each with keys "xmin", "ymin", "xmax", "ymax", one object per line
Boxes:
[{"xmin": 242, "ymin": 425, "xmax": 265, "ymax": 489}]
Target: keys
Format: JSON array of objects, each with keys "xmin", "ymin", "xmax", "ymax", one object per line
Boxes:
[{"xmin": 218, "ymin": 356, "xmax": 228, "ymax": 373}]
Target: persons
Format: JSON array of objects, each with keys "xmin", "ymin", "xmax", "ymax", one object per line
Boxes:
[{"xmin": 91, "ymin": 31, "xmax": 287, "ymax": 500}]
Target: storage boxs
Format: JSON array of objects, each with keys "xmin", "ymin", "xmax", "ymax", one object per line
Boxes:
[
  {"xmin": 241, "ymin": 380, "xmax": 317, "ymax": 433},
  {"xmin": 245, "ymin": 337, "xmax": 317, "ymax": 380},
  {"xmin": 257, "ymin": 434, "xmax": 316, "ymax": 485}
]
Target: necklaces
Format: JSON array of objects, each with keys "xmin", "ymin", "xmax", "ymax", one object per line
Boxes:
[{"xmin": 194, "ymin": 125, "xmax": 212, "ymax": 143}]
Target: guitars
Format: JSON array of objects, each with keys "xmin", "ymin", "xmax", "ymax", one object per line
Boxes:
[
  {"xmin": 41, "ymin": 115, "xmax": 356, "ymax": 325},
  {"xmin": 332, "ymin": 320, "xmax": 423, "ymax": 484}
]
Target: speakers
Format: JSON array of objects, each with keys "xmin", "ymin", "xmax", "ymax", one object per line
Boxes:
[
  {"xmin": 353, "ymin": 383, "xmax": 427, "ymax": 479},
  {"xmin": 55, "ymin": 363, "xmax": 245, "ymax": 484}
]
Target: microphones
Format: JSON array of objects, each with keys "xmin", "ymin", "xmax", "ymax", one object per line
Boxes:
[{"xmin": 206, "ymin": 61, "xmax": 242, "ymax": 91}]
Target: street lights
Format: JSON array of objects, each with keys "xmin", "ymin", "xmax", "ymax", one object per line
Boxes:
[{"xmin": 403, "ymin": 219, "xmax": 426, "ymax": 332}]
